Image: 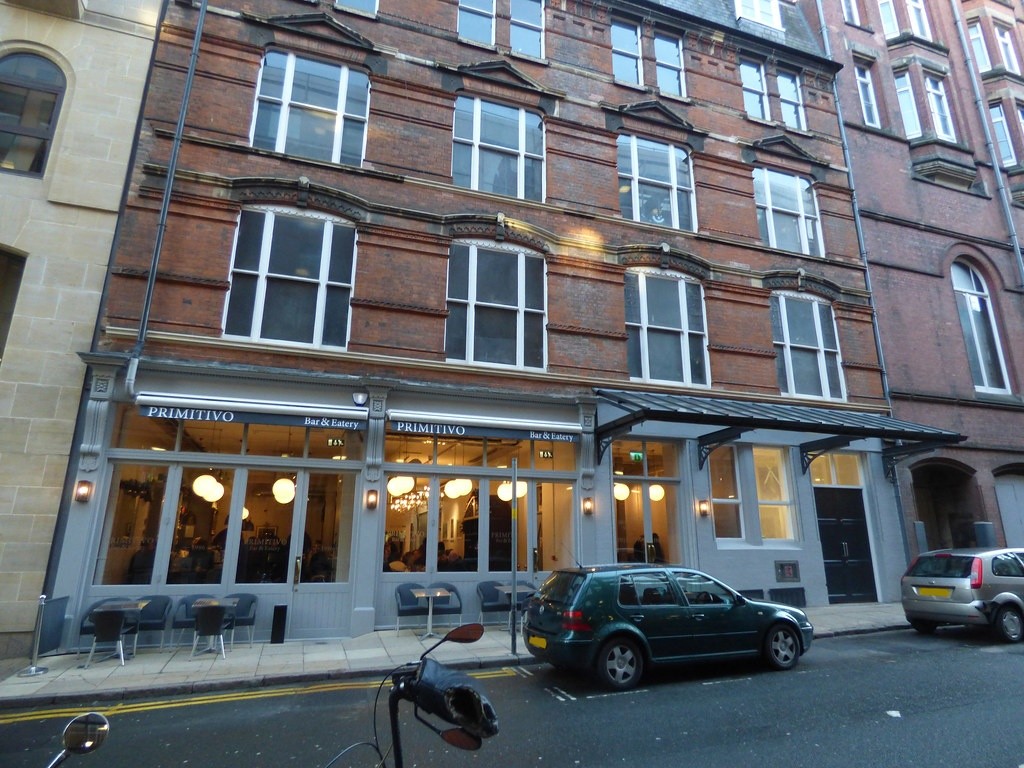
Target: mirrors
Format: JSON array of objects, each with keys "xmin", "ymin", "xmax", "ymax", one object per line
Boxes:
[{"xmin": 109, "ymin": 488, "xmax": 140, "ymax": 546}]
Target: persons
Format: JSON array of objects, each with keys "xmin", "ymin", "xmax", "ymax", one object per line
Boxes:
[
  {"xmin": 412, "ymin": 539, "xmax": 465, "ymax": 571},
  {"xmin": 634, "ymin": 533, "xmax": 664, "ymax": 562}
]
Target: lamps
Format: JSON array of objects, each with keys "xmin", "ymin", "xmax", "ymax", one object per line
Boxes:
[
  {"xmin": 699, "ymin": 500, "xmax": 710, "ymax": 517},
  {"xmin": 193, "ymin": 422, "xmax": 225, "ymax": 502},
  {"xmin": 387, "ymin": 436, "xmax": 415, "ymax": 497},
  {"xmin": 272, "ymin": 426, "xmax": 295, "ymax": 504},
  {"xmin": 513, "ymin": 440, "xmax": 527, "ymax": 499},
  {"xmin": 583, "ymin": 497, "xmax": 593, "ymax": 515},
  {"xmin": 74, "ymin": 480, "xmax": 92, "ymax": 502},
  {"xmin": 367, "ymin": 490, "xmax": 377, "ymax": 508},
  {"xmin": 649, "ymin": 449, "xmax": 665, "ymax": 501},
  {"xmin": 444, "ymin": 439, "xmax": 473, "ymax": 498},
  {"xmin": 497, "ymin": 479, "xmax": 513, "ymax": 502},
  {"xmin": 614, "ymin": 447, "xmax": 630, "ymax": 501},
  {"xmin": 352, "ymin": 393, "xmax": 368, "ymax": 406}
]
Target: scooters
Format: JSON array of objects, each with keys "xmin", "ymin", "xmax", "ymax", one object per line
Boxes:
[{"xmin": 325, "ymin": 623, "xmax": 500, "ymax": 768}]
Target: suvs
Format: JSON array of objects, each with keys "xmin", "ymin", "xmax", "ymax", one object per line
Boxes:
[
  {"xmin": 522, "ymin": 562, "xmax": 814, "ymax": 690},
  {"xmin": 901, "ymin": 547, "xmax": 1024, "ymax": 643}
]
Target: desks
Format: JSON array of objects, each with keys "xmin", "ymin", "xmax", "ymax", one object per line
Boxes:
[
  {"xmin": 495, "ymin": 585, "xmax": 536, "ymax": 631},
  {"xmin": 191, "ymin": 598, "xmax": 239, "ymax": 656},
  {"xmin": 409, "ymin": 587, "xmax": 452, "ymax": 641},
  {"xmin": 94, "ymin": 600, "xmax": 151, "ymax": 663}
]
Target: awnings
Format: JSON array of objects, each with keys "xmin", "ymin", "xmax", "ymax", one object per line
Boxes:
[{"xmin": 595, "ymin": 389, "xmax": 970, "ymax": 476}]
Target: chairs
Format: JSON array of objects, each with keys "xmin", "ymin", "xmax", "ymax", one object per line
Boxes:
[
  {"xmin": 642, "ymin": 588, "xmax": 664, "ymax": 605},
  {"xmin": 476, "ymin": 580, "xmax": 537, "ymax": 634},
  {"xmin": 395, "ymin": 581, "xmax": 462, "ymax": 638},
  {"xmin": 77, "ymin": 593, "xmax": 258, "ymax": 670}
]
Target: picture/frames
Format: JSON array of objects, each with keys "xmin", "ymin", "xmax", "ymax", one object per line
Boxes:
[{"xmin": 255, "ymin": 526, "xmax": 278, "ymax": 539}]
